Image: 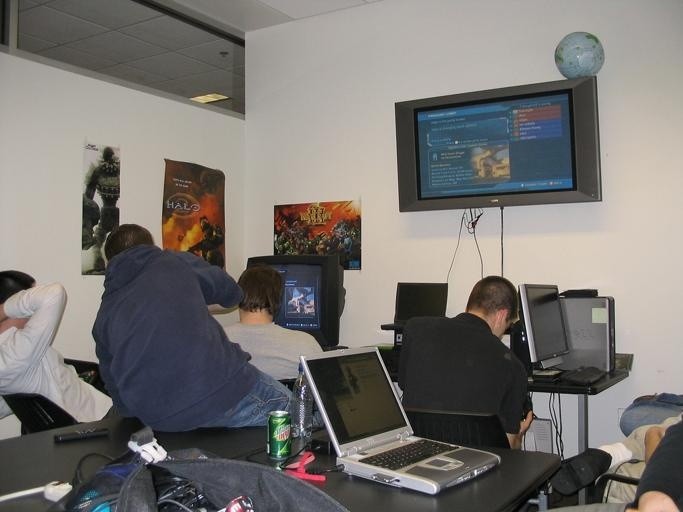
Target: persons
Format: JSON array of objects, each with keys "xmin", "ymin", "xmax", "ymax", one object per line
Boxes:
[
  {"xmin": 0, "ymin": 271, "xmax": 115, "ymax": 427},
  {"xmin": 214, "ymin": 264, "xmax": 322, "ymax": 381},
  {"xmin": 394, "ymin": 274, "xmax": 533, "ymax": 450},
  {"xmin": 551, "ymin": 410, "xmax": 683, "ymax": 505},
  {"xmin": 623, "ymin": 420, "xmax": 683, "ymax": 512},
  {"xmin": 90, "ymin": 224, "xmax": 296, "ymax": 433}
]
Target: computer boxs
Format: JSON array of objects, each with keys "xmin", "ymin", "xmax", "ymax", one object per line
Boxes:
[{"xmin": 550, "ymin": 297, "xmax": 616, "ymax": 373}]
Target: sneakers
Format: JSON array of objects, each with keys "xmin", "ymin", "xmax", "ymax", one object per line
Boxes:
[{"xmin": 546, "ymin": 447, "xmax": 613, "ymax": 496}]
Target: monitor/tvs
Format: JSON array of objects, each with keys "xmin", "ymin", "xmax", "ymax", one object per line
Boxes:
[
  {"xmin": 244, "ymin": 253, "xmax": 346, "ymax": 349},
  {"xmin": 394, "ymin": 75, "xmax": 604, "ymax": 213},
  {"xmin": 518, "ymin": 284, "xmax": 571, "ymax": 385}
]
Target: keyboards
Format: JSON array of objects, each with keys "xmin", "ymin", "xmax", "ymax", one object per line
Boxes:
[{"xmin": 558, "ymin": 366, "xmax": 608, "ymax": 386}]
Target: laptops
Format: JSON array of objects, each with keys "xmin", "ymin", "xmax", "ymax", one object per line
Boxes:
[
  {"xmin": 381, "ymin": 283, "xmax": 448, "ymax": 330},
  {"xmin": 298, "ymin": 346, "xmax": 501, "ymax": 495}
]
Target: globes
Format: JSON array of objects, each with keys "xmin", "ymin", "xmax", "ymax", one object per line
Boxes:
[{"xmin": 553, "ymin": 31, "xmax": 606, "ymax": 81}]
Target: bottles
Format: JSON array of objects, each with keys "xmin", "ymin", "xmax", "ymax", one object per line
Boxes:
[
  {"xmin": 539, "ymin": 490, "xmax": 547, "ymax": 512},
  {"xmin": 291, "ymin": 363, "xmax": 312, "ymax": 437},
  {"xmin": 266, "ymin": 411, "xmax": 291, "ymax": 460}
]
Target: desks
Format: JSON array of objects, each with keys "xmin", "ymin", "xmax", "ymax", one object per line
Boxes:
[
  {"xmin": 1, "ymin": 415, "xmax": 562, "ymax": 512},
  {"xmin": 360, "ymin": 345, "xmax": 634, "ymax": 507}
]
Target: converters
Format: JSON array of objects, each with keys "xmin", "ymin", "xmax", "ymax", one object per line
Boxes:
[{"xmin": 305, "ymin": 435, "xmax": 337, "ymax": 456}]
